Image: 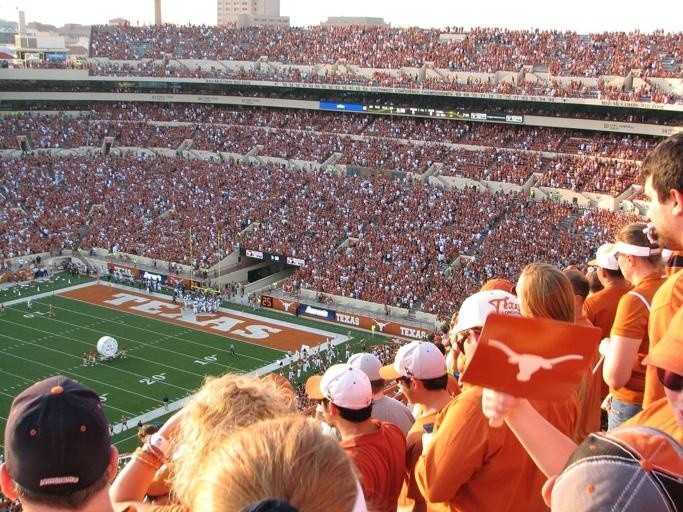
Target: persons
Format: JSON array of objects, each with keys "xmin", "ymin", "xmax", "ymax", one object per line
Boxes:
[{"xmin": 0, "ymin": 21, "xmax": 681, "ymax": 512}]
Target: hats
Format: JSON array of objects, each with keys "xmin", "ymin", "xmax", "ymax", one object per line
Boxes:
[
  {"xmin": 587, "ymin": 241, "xmax": 661, "ymax": 271},
  {"xmin": 4, "ymin": 375, "xmax": 110, "ymax": 494},
  {"xmin": 543, "ymin": 425, "xmax": 683, "ymax": 512},
  {"xmin": 305, "ymin": 339, "xmax": 448, "ymax": 410},
  {"xmin": 452, "ymin": 278, "xmax": 521, "ymax": 334}
]
